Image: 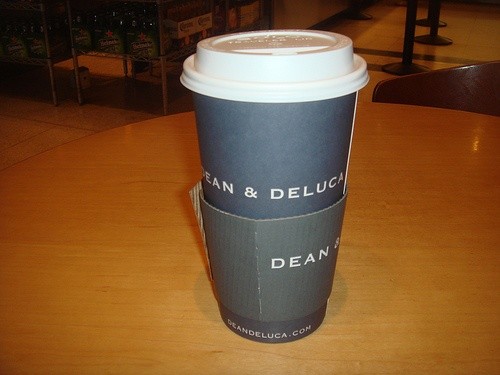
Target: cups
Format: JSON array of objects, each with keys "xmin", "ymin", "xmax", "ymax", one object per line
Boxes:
[{"xmin": 179, "ymin": 29, "xmax": 370, "ymax": 343}]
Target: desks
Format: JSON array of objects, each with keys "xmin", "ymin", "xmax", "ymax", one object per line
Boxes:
[{"xmin": 0, "ymin": 102, "xmax": 500, "ymax": 375}]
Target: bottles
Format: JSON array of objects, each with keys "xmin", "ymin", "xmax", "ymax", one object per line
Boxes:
[
  {"xmin": 0, "ymin": 21, "xmax": 27, "ymax": 60},
  {"xmin": 22, "ymin": 24, "xmax": 44, "ymax": 57},
  {"xmin": 72, "ymin": 3, "xmax": 158, "ymax": 58},
  {"xmin": 47, "ymin": 16, "xmax": 70, "ymax": 58}
]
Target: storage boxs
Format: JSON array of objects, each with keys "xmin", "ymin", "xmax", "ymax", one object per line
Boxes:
[{"xmin": 164, "ymin": 2, "xmax": 213, "ymax": 39}]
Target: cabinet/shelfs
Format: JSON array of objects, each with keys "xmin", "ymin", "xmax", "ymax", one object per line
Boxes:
[
  {"xmin": 0, "ymin": 0, "xmax": 97, "ymax": 106},
  {"xmin": 66, "ymin": 0, "xmax": 272, "ymax": 115}
]
